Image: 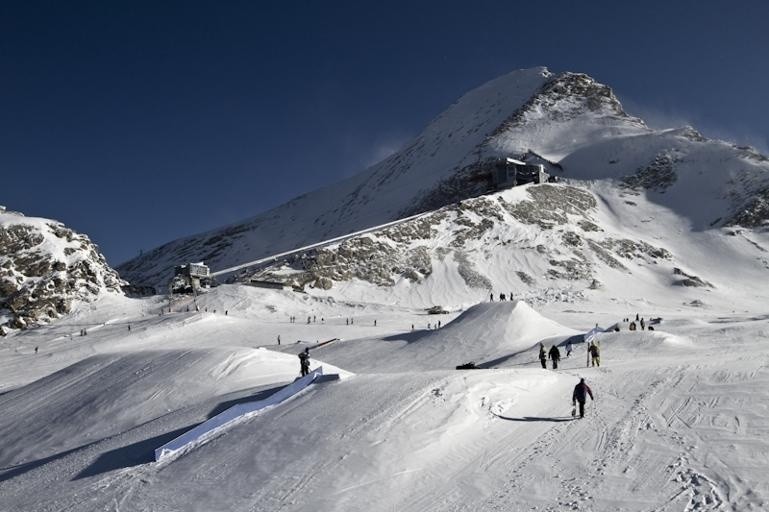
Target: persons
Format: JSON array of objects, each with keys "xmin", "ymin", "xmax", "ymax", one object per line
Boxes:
[
  {"xmin": 614, "ymin": 311, "xmax": 655, "ymax": 332},
  {"xmin": 571, "ymin": 377, "xmax": 594, "ymax": 419},
  {"xmin": 564, "ymin": 339, "xmax": 572, "ymax": 357},
  {"xmin": 587, "ymin": 339, "xmax": 600, "ymax": 368},
  {"xmin": 298, "ymin": 347, "xmax": 310, "ymax": 377},
  {"xmin": 538, "ymin": 342, "xmax": 547, "ymax": 369},
  {"xmin": 548, "ymin": 344, "xmax": 560, "ymax": 369}
]
